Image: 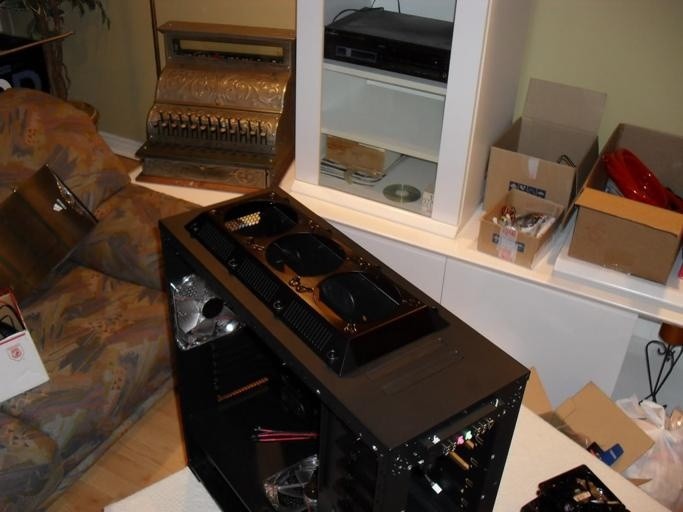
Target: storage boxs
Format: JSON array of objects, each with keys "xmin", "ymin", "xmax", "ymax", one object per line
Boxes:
[
  {"xmin": 478, "ymin": 189, "xmax": 564, "ymax": 269},
  {"xmin": 569, "ymin": 124, "xmax": 683, "ymax": 285},
  {"xmin": 522, "ymin": 366, "xmax": 655, "ymax": 473},
  {"xmin": 0, "ymin": 292, "xmax": 50, "ymax": 401},
  {"xmin": 482, "ymin": 78, "xmax": 607, "ymax": 230},
  {"xmin": 326, "ymin": 134, "xmax": 402, "ymax": 178}
]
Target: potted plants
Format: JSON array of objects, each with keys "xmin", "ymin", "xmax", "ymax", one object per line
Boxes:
[{"xmin": 0, "ymin": 0, "xmax": 111, "ymax": 134}]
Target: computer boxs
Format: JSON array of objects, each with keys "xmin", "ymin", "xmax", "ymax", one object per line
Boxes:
[{"xmin": 156, "ymin": 184, "xmax": 532, "ymax": 510}]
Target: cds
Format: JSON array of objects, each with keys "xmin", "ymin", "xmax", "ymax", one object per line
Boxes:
[{"xmin": 383, "ymin": 183, "xmax": 421, "ymax": 203}]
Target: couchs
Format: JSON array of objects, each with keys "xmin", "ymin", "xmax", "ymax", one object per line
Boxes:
[{"xmin": 0, "ymin": 88, "xmax": 206, "ymax": 512}]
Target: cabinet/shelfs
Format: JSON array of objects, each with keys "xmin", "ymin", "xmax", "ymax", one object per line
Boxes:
[{"xmin": 279, "ymin": 194, "xmax": 683, "ymax": 415}]
